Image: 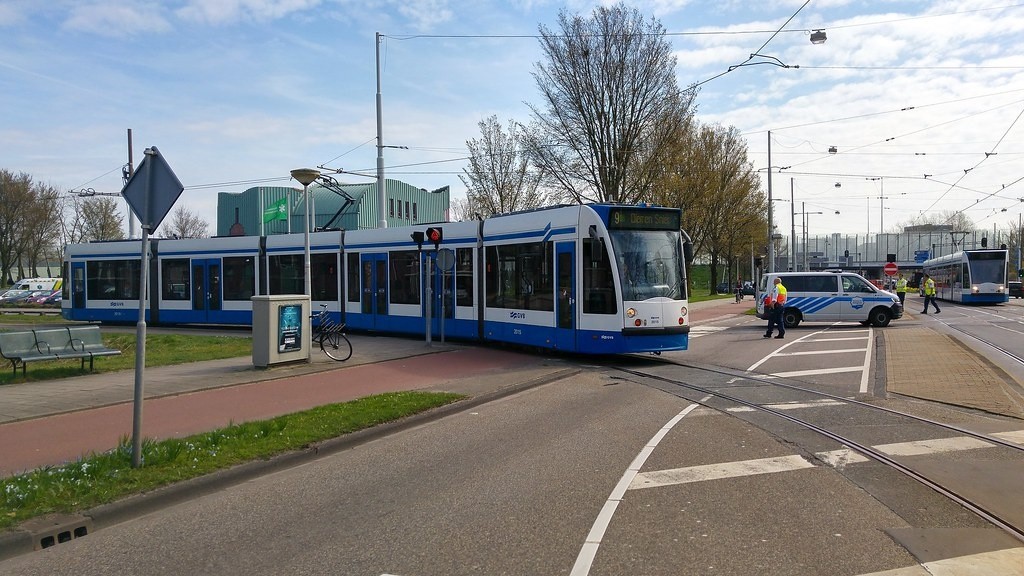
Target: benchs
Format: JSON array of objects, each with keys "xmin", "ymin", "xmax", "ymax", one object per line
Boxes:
[{"xmin": 0, "ymin": 325, "xmax": 122, "ymax": 378}]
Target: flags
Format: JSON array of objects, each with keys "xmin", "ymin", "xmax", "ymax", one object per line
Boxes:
[{"xmin": 262, "ymin": 198, "xmax": 288, "ymax": 223}]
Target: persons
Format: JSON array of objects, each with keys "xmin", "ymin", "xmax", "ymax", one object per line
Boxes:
[
  {"xmin": 894, "ymin": 273, "xmax": 907, "ymax": 313},
  {"xmin": 920, "ymin": 272, "xmax": 942, "ymax": 314},
  {"xmin": 734, "ymin": 279, "xmax": 744, "ymax": 302},
  {"xmin": 763, "ymin": 277, "xmax": 787, "ymax": 338},
  {"xmin": 752, "ymin": 282, "xmax": 756, "ymax": 298}
]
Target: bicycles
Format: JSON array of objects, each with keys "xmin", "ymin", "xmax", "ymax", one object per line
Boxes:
[
  {"xmin": 736, "ymin": 288, "xmax": 745, "ymax": 304},
  {"xmin": 307, "ymin": 305, "xmax": 353, "ymax": 361}
]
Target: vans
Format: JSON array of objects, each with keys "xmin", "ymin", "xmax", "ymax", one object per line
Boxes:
[
  {"xmin": 756, "ymin": 270, "xmax": 904, "ymax": 329},
  {"xmin": 9, "ymin": 278, "xmax": 62, "ymax": 292}
]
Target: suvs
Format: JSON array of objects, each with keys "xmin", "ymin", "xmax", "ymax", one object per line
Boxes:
[{"xmin": 869, "ymin": 280, "xmax": 884, "ymax": 289}]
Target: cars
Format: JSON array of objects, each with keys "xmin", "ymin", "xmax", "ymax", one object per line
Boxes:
[
  {"xmin": 0, "ymin": 290, "xmax": 26, "ymax": 306},
  {"xmin": 715, "ymin": 282, "xmax": 755, "ymax": 295},
  {"xmin": 27, "ymin": 290, "xmax": 62, "ymax": 306},
  {"xmin": 47, "ymin": 292, "xmax": 62, "ymax": 305},
  {"xmin": 884, "ymin": 279, "xmax": 898, "ymax": 290},
  {"xmin": 3, "ymin": 290, "xmax": 45, "ymax": 306}
]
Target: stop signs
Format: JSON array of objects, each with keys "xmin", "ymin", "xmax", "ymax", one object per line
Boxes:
[{"xmin": 884, "ymin": 262, "xmax": 899, "ymax": 276}]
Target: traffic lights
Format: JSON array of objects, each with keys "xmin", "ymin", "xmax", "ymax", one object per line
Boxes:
[
  {"xmin": 1019, "ymin": 269, "xmax": 1024, "ymax": 277},
  {"xmin": 981, "ymin": 238, "xmax": 987, "ymax": 247},
  {"xmin": 425, "ymin": 227, "xmax": 443, "ymax": 244}
]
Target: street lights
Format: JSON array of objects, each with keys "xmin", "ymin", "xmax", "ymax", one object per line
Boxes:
[
  {"xmin": 769, "ymin": 233, "xmax": 784, "ymax": 273},
  {"xmin": 289, "ymin": 168, "xmax": 321, "ymax": 305},
  {"xmin": 794, "ymin": 211, "xmax": 823, "ymax": 272},
  {"xmin": 735, "ymin": 255, "xmax": 740, "ymax": 283}
]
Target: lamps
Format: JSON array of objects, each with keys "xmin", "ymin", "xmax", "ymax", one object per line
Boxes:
[
  {"xmin": 834, "ymin": 182, "xmax": 842, "ymax": 188},
  {"xmin": 829, "ymin": 146, "xmax": 838, "ymax": 154},
  {"xmin": 810, "ymin": 29, "xmax": 828, "ymax": 46}
]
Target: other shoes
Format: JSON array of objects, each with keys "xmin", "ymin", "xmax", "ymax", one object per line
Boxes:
[
  {"xmin": 934, "ymin": 309, "xmax": 941, "ymax": 314},
  {"xmin": 763, "ymin": 335, "xmax": 771, "ymax": 338},
  {"xmin": 774, "ymin": 336, "xmax": 784, "ymax": 339},
  {"xmin": 920, "ymin": 312, "xmax": 927, "ymax": 314}
]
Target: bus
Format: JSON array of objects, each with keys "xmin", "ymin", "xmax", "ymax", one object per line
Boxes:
[
  {"xmin": 62, "ymin": 168, "xmax": 693, "ymax": 359},
  {"xmin": 923, "ymin": 230, "xmax": 1011, "ymax": 306}
]
